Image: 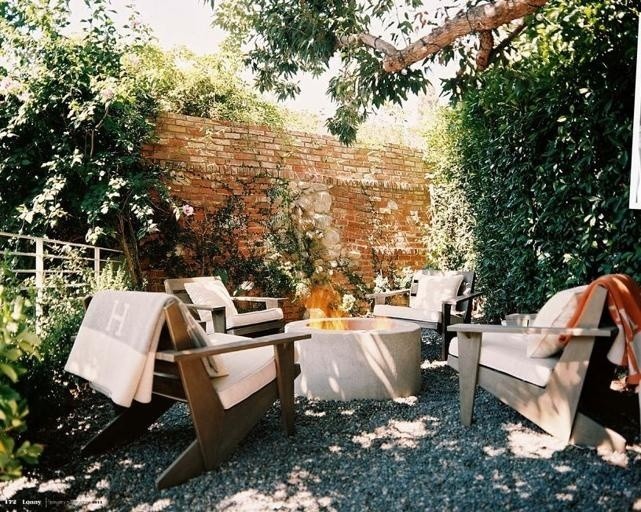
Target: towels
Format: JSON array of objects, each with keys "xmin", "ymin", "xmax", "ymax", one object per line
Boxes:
[
  {"xmin": 55, "ymin": 286, "xmax": 229, "ymax": 409},
  {"xmin": 560, "ymin": 274, "xmax": 641, "ymax": 388}
]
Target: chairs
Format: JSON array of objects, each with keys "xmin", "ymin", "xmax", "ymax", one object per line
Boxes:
[{"xmin": 82, "ymin": 268, "xmax": 626, "ymax": 494}]
